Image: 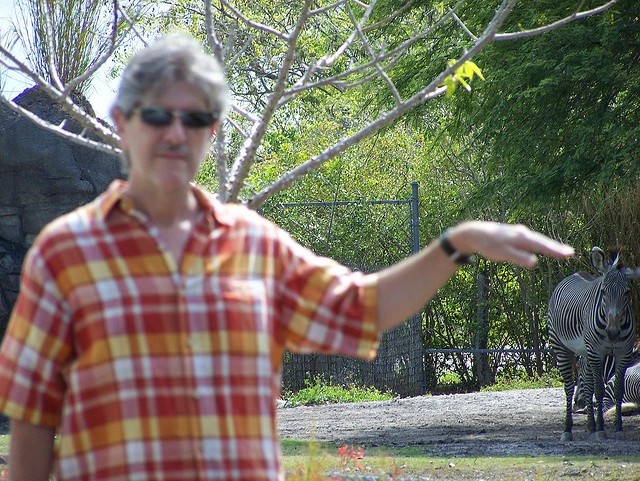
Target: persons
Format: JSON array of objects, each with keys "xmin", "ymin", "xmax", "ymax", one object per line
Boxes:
[{"xmin": 0, "ymin": 32, "xmax": 579, "ymax": 480}]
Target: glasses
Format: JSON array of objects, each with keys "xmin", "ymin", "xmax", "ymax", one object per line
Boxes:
[{"xmin": 133, "ymin": 106, "xmax": 220, "ymax": 126}]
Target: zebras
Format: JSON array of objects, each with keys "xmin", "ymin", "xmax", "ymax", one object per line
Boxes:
[
  {"xmin": 573, "ymin": 347, "xmax": 640, "ymax": 413},
  {"xmin": 546, "ymin": 245, "xmax": 639, "ymax": 442}
]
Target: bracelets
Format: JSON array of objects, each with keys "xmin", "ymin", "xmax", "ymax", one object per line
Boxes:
[{"xmin": 434, "ymin": 226, "xmax": 469, "ymax": 269}]
